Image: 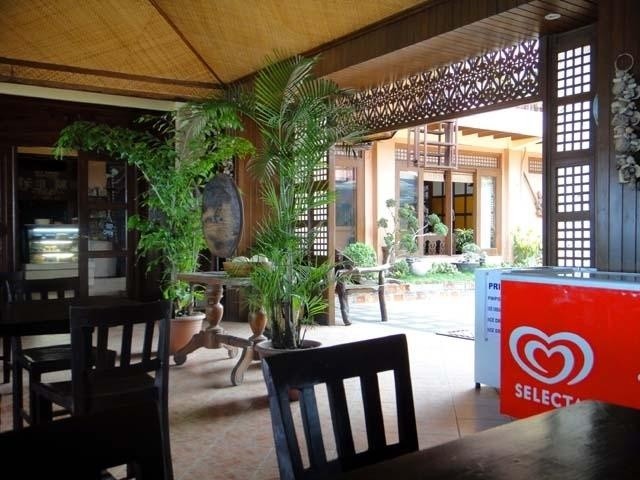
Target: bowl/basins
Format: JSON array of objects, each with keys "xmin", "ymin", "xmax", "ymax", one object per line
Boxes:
[{"xmin": 233, "ymin": 253, "xmax": 269, "ymax": 262}]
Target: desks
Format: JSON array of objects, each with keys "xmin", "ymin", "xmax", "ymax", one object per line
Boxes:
[
  {"xmin": 1, "ymin": 291, "xmax": 164, "ymax": 338},
  {"xmin": 167, "ymin": 268, "xmax": 287, "ymax": 385},
  {"xmin": 313, "ymin": 398, "xmax": 640, "ymax": 480}
]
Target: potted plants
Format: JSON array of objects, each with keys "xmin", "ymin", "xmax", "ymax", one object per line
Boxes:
[
  {"xmin": 198, "ymin": 47, "xmax": 378, "ymax": 402},
  {"xmin": 452, "ymin": 227, "xmax": 481, "ymax": 255},
  {"xmin": 49, "ymin": 92, "xmax": 257, "ymax": 354}
]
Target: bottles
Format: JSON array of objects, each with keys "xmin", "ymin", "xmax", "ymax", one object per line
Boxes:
[{"xmin": 102, "ymin": 210, "xmax": 114, "ymax": 237}]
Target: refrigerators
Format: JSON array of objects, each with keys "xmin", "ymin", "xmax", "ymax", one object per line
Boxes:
[{"xmin": 498, "ymin": 267, "xmax": 640, "ymax": 421}]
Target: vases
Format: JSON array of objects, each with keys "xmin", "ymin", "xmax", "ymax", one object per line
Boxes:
[{"xmin": 452, "ymin": 260, "xmax": 480, "ymax": 274}]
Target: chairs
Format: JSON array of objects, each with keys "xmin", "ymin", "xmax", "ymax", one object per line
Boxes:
[
  {"xmin": 255, "ymin": 331, "xmax": 419, "ymax": 479},
  {"xmin": 1, "ymin": 394, "xmax": 176, "ymax": 479},
  {"xmin": 31, "ymin": 298, "xmax": 174, "ymax": 478},
  {"xmin": 9, "ymin": 276, "xmax": 112, "ymax": 426}
]
